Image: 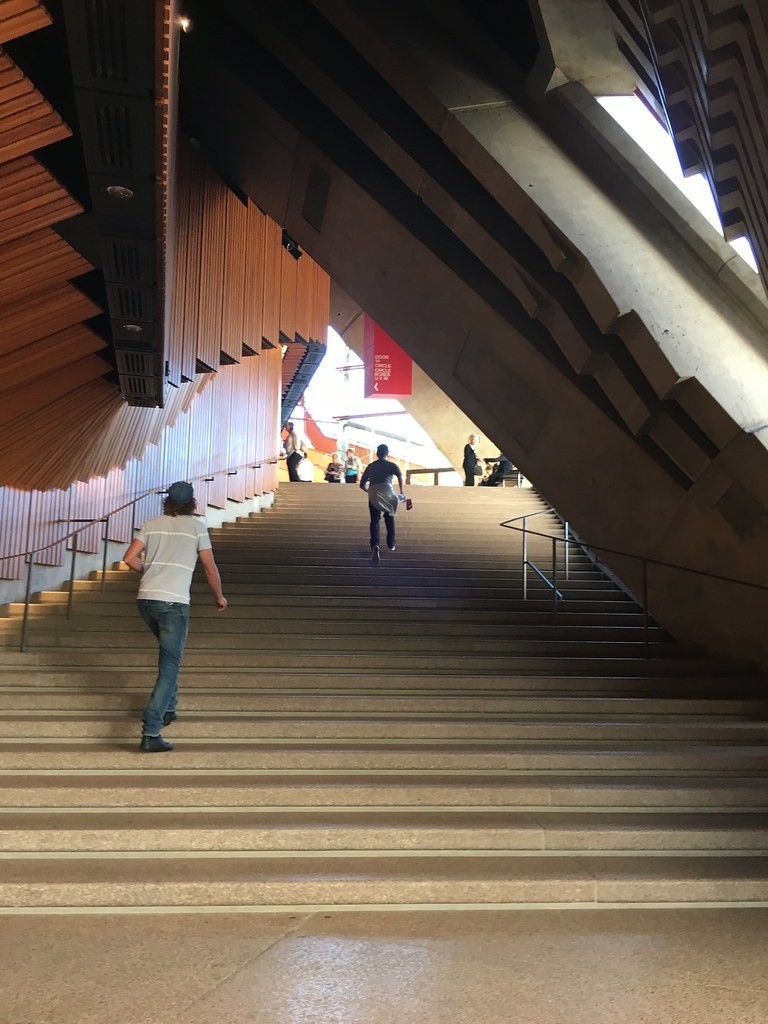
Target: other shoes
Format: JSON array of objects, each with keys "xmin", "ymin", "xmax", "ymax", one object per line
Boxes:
[
  {"xmin": 140, "ymin": 734, "xmax": 174, "ymax": 752},
  {"xmin": 163, "ymin": 711, "xmax": 176, "ymax": 726},
  {"xmin": 389, "ymin": 545, "xmax": 395, "ymax": 551},
  {"xmin": 372, "ymin": 545, "xmax": 380, "ymax": 566}
]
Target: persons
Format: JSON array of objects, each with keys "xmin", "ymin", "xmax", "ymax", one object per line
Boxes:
[
  {"xmin": 297, "ymin": 453, "xmax": 314, "ymax": 482},
  {"xmin": 463, "ymin": 434, "xmax": 482, "ymax": 486},
  {"xmin": 343, "ymin": 447, "xmax": 362, "ymax": 483},
  {"xmin": 284, "ymin": 422, "xmax": 303, "ymax": 482},
  {"xmin": 478, "ymin": 476, "xmax": 489, "ymax": 486},
  {"xmin": 325, "ymin": 454, "xmax": 343, "ymax": 483},
  {"xmin": 124, "ymin": 482, "xmax": 228, "ymax": 751},
  {"xmin": 484, "ymin": 450, "xmax": 512, "ymax": 487},
  {"xmin": 360, "ymin": 444, "xmax": 405, "ymax": 562}
]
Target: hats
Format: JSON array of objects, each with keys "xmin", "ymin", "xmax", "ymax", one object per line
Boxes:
[{"xmin": 168, "ymin": 481, "xmax": 192, "ymax": 503}]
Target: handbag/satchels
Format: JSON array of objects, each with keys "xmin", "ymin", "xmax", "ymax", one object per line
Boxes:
[
  {"xmin": 324, "ymin": 463, "xmax": 333, "ymax": 480},
  {"xmin": 473, "ymin": 460, "xmax": 483, "ymax": 476}
]
